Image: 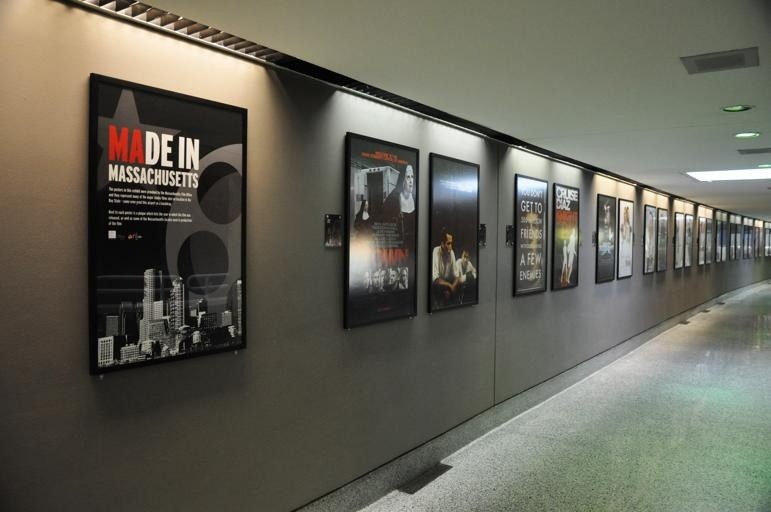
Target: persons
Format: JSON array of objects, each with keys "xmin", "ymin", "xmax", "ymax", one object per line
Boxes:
[
  {"xmin": 384, "ymin": 164, "xmax": 415, "ymax": 214},
  {"xmin": 432, "ymin": 232, "xmax": 459, "ymax": 299},
  {"xmin": 355, "ymin": 200, "xmax": 370, "ymax": 222},
  {"xmin": 457, "ymin": 248, "xmax": 477, "ymax": 283}
]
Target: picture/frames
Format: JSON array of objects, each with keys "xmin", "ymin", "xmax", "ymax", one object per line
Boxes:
[
  {"xmin": 340, "ymin": 127, "xmax": 483, "ymax": 328},
  {"xmin": 642, "ymin": 203, "xmax": 669, "ymax": 274},
  {"xmin": 595, "ymin": 193, "xmax": 635, "ymax": 283},
  {"xmin": 512, "ymin": 168, "xmax": 582, "ymax": 296},
  {"xmin": 674, "ymin": 211, "xmax": 770, "ymax": 272},
  {"xmin": 84, "ymin": 70, "xmax": 249, "ymax": 375}
]
